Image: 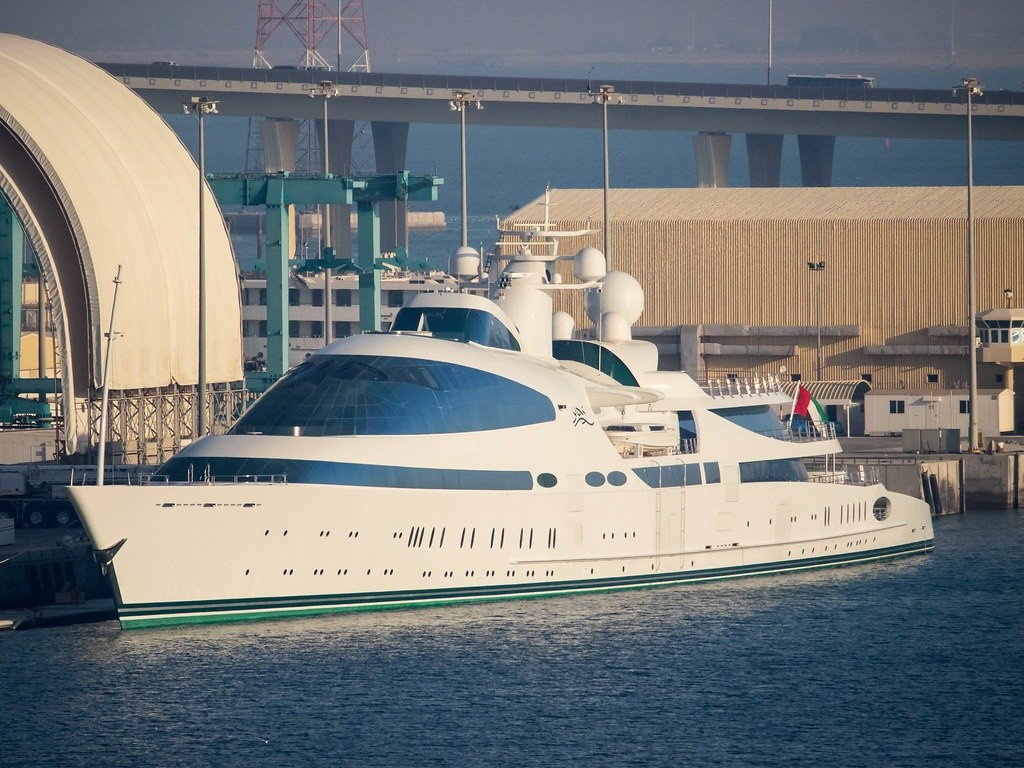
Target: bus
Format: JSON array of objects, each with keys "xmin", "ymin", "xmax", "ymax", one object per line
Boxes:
[{"xmin": 789, "ymin": 75, "xmax": 877, "ymax": 90}]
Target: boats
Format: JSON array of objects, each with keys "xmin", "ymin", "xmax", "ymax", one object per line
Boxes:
[{"xmin": 63, "ymin": 182, "xmax": 935, "ymax": 629}]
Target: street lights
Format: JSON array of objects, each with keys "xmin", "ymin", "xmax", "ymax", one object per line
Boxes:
[
  {"xmin": 807, "ymin": 262, "xmax": 825, "ymax": 382},
  {"xmin": 182, "ymin": 95, "xmax": 221, "ymax": 437},
  {"xmin": 307, "ymin": 78, "xmax": 340, "ymax": 346},
  {"xmin": 951, "ymin": 77, "xmax": 983, "ymax": 454},
  {"xmin": 586, "ymin": 83, "xmax": 623, "ymax": 273},
  {"xmin": 448, "ymin": 88, "xmax": 484, "ymax": 247}
]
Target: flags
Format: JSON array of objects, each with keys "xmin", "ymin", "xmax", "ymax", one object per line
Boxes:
[
  {"xmin": 807, "ymin": 396, "xmax": 830, "ymax": 431},
  {"xmin": 794, "ymin": 386, "xmax": 812, "ymax": 418}
]
